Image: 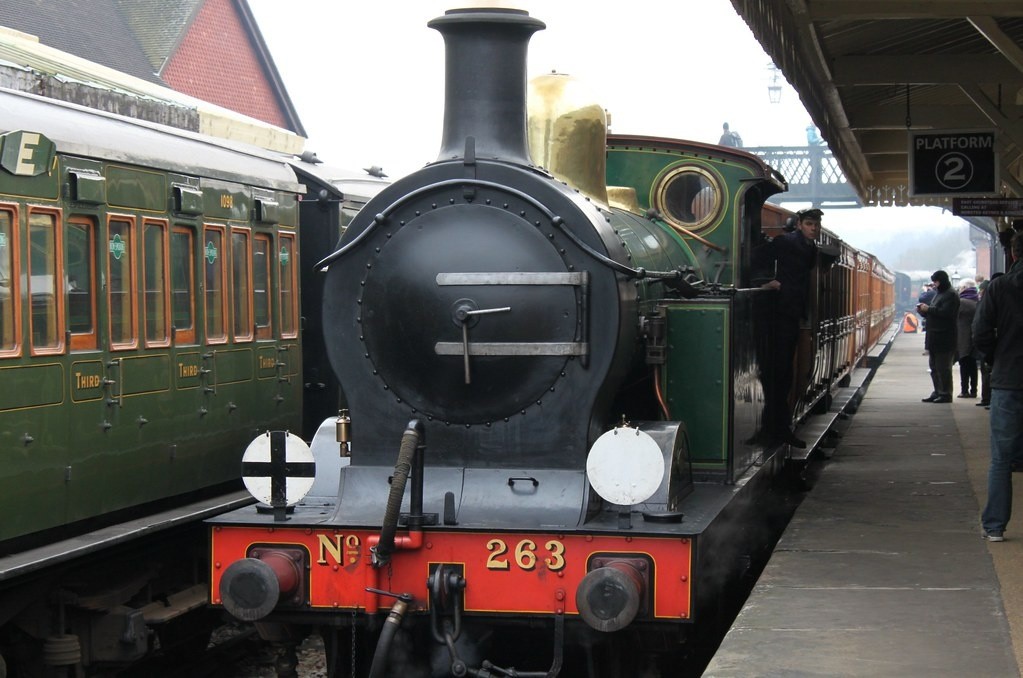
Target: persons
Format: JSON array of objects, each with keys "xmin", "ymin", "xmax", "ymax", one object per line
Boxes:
[
  {"xmin": 717, "ymin": 121, "xmax": 736, "ymax": 146},
  {"xmin": 917, "ymin": 276, "xmax": 984, "ymax": 356},
  {"xmin": 902, "ymin": 308, "xmax": 918, "ymax": 333},
  {"xmin": 975, "ymin": 279, "xmax": 992, "ymax": 406},
  {"xmin": 753, "ymin": 206, "xmax": 825, "ymax": 450},
  {"xmin": 955, "ymin": 278, "xmax": 980, "ymax": 399},
  {"xmin": 984, "ymin": 271, "xmax": 1004, "ymax": 410},
  {"xmin": 969, "ymin": 230, "xmax": 1023, "ymax": 543},
  {"xmin": 914, "ymin": 271, "xmax": 959, "ymax": 405}
]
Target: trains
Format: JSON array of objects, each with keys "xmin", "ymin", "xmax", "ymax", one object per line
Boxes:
[
  {"xmin": 197, "ymin": 4, "xmax": 930, "ymax": 678},
  {"xmin": 0, "ymin": 18, "xmax": 392, "ymax": 606}
]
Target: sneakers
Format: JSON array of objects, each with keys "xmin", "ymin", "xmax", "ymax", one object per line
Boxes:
[
  {"xmin": 975, "ymin": 402, "xmax": 990, "ymax": 406},
  {"xmin": 984, "ymin": 405, "xmax": 990, "ymax": 410},
  {"xmin": 981, "ymin": 531, "xmax": 1004, "ymax": 542},
  {"xmin": 957, "ymin": 392, "xmax": 969, "ymax": 398},
  {"xmin": 965, "ymin": 392, "xmax": 977, "ymax": 398}
]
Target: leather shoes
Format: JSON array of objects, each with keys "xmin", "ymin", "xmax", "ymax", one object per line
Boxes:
[
  {"xmin": 933, "ymin": 396, "xmax": 952, "ymax": 403},
  {"xmin": 922, "ymin": 397, "xmax": 937, "ymax": 402},
  {"xmin": 777, "ymin": 426, "xmax": 807, "ymax": 450}
]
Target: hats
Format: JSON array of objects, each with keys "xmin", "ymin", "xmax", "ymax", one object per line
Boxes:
[
  {"xmin": 796, "ymin": 208, "xmax": 825, "ymax": 218},
  {"xmin": 931, "ymin": 271, "xmax": 948, "ymax": 281}
]
